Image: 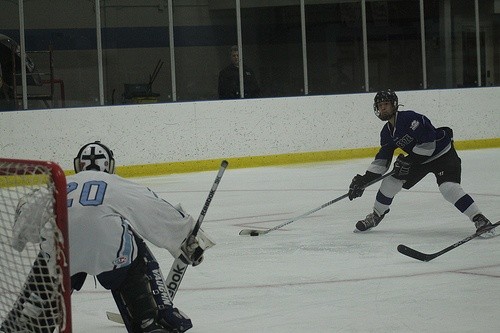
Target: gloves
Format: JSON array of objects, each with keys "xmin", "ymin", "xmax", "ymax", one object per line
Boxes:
[
  {"xmin": 182, "ymin": 233, "xmax": 199, "ymax": 258},
  {"xmin": 348, "ymin": 173, "xmax": 365, "ymax": 201},
  {"xmin": 391, "ymin": 153, "xmax": 412, "ymax": 180}
]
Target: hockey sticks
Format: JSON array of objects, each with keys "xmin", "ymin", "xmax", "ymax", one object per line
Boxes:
[
  {"xmin": 397, "ymin": 221, "xmax": 500, "ymax": 262},
  {"xmin": 106, "ymin": 160, "xmax": 229, "ymax": 324},
  {"xmin": 238, "ymin": 171, "xmax": 395, "ymax": 235}
]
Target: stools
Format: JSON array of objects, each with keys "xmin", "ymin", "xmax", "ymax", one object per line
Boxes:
[{"xmin": 122, "ymin": 83, "xmax": 160, "ymax": 105}]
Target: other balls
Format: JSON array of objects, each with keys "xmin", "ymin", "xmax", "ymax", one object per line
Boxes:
[{"xmin": 250, "ymin": 232, "xmax": 259, "ymax": 236}]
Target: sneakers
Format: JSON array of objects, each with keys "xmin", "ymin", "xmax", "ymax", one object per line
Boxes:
[
  {"xmin": 353, "ymin": 209, "xmax": 390, "ymax": 234},
  {"xmin": 473, "ymin": 214, "xmax": 496, "ymax": 238}
]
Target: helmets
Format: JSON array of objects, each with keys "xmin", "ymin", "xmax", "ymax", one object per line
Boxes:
[
  {"xmin": 74, "ymin": 141, "xmax": 116, "ymax": 174},
  {"xmin": 374, "ymin": 89, "xmax": 399, "ymax": 121}
]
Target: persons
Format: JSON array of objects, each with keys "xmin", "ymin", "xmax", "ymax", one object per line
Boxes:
[
  {"xmin": 0, "ymin": 139, "xmax": 216, "ymax": 333},
  {"xmin": 348, "ymin": 89, "xmax": 494, "ymax": 234},
  {"xmin": 217, "ymin": 46, "xmax": 256, "ymax": 100}
]
